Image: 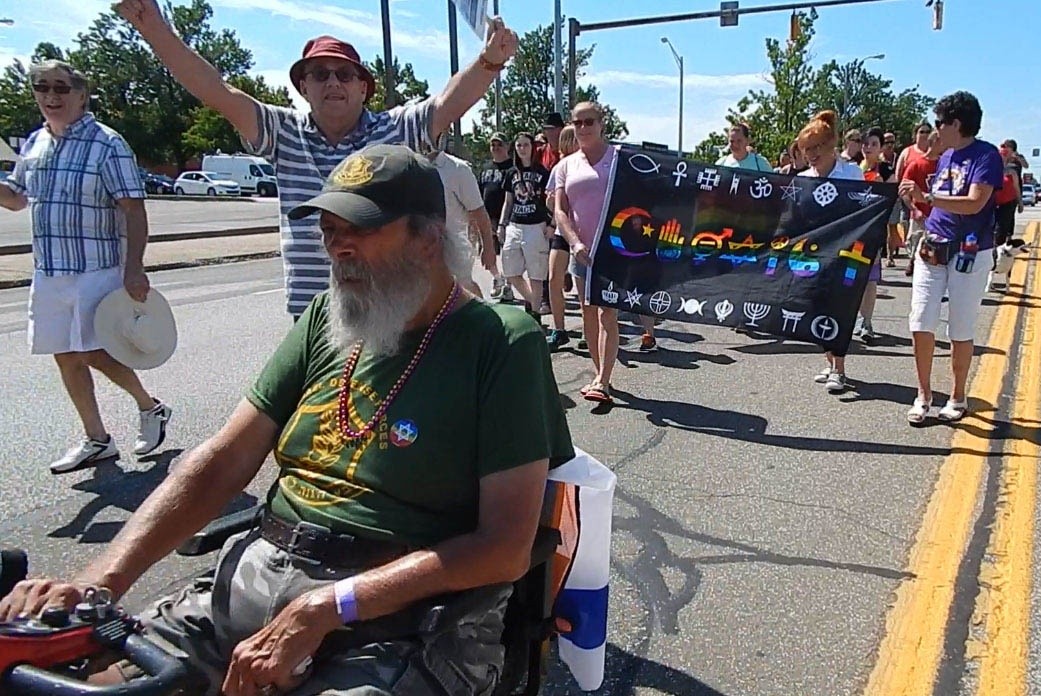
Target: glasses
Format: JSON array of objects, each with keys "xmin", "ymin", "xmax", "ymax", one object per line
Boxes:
[
  {"xmin": 304, "ymin": 66, "xmax": 358, "ymax": 83},
  {"xmin": 572, "ymin": 119, "xmax": 596, "ymax": 127},
  {"xmin": 918, "ymin": 130, "xmax": 931, "ymax": 134},
  {"xmin": 884, "ymin": 141, "xmax": 896, "ymax": 145},
  {"xmin": 33, "ymin": 84, "xmax": 81, "ymax": 94},
  {"xmin": 851, "ymin": 139, "xmax": 860, "ymax": 143},
  {"xmin": 934, "ymin": 121, "xmax": 944, "ymax": 129}
]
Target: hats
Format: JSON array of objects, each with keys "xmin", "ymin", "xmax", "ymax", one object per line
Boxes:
[
  {"xmin": 490, "ymin": 131, "xmax": 508, "ymax": 143},
  {"xmin": 95, "ymin": 287, "xmax": 178, "ymax": 370},
  {"xmin": 540, "ymin": 112, "xmax": 564, "ymax": 127},
  {"xmin": 288, "ymin": 142, "xmax": 446, "ymax": 228},
  {"xmin": 290, "ymin": 37, "xmax": 375, "ymax": 104}
]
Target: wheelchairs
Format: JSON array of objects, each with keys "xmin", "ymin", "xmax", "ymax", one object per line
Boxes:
[{"xmin": 0, "ymin": 478, "xmax": 580, "ymax": 696}]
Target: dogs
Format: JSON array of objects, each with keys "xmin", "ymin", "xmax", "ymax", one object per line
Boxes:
[{"xmin": 984, "ymin": 236, "xmax": 1031, "ymax": 293}]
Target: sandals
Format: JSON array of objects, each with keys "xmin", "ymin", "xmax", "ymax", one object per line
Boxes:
[
  {"xmin": 907, "ymin": 395, "xmax": 933, "ymax": 424},
  {"xmin": 937, "ymin": 395, "xmax": 968, "ymax": 421}
]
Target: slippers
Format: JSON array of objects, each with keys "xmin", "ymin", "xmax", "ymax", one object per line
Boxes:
[
  {"xmin": 581, "ymin": 383, "xmax": 591, "ymax": 394},
  {"xmin": 584, "ymin": 388, "xmax": 613, "ymax": 402}
]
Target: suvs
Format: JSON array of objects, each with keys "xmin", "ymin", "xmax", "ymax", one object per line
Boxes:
[{"xmin": 1022, "ymin": 184, "xmax": 1036, "ymax": 206}]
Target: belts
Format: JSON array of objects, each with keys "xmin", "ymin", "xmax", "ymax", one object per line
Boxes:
[{"xmin": 259, "ymin": 512, "xmax": 405, "ymax": 570}]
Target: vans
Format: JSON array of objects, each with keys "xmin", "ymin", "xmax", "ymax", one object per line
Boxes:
[{"xmin": 202, "ymin": 153, "xmax": 277, "ymax": 197}]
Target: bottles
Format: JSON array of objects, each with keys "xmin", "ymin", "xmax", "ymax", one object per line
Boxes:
[{"xmin": 955, "ymin": 234, "xmax": 978, "ymax": 273}]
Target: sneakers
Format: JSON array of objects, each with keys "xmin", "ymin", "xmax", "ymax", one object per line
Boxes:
[
  {"xmin": 49, "ymin": 434, "xmax": 119, "ymax": 473},
  {"xmin": 134, "ymin": 398, "xmax": 172, "ymax": 455},
  {"xmin": 815, "ymin": 368, "xmax": 832, "ymax": 382},
  {"xmin": 825, "ymin": 373, "xmax": 846, "ymax": 390},
  {"xmin": 490, "ymin": 272, "xmax": 573, "ymax": 325},
  {"xmin": 640, "ymin": 335, "xmax": 657, "ymax": 351}
]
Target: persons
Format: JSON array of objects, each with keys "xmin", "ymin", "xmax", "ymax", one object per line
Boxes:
[
  {"xmin": 897, "ymin": 90, "xmax": 1005, "ymax": 425},
  {"xmin": 1, "ymin": 145, "xmax": 574, "ymax": 696},
  {"xmin": 0, "ymin": 60, "xmax": 174, "ymax": 474},
  {"xmin": 107, "ymin": 0, "xmax": 1028, "ymax": 402}
]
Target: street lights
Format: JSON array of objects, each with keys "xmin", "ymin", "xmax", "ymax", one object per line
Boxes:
[
  {"xmin": 661, "ymin": 38, "xmax": 683, "ymax": 159},
  {"xmin": 842, "ymin": 54, "xmax": 885, "ymax": 150}
]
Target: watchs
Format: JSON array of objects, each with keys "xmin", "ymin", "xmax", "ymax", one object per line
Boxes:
[{"xmin": 923, "ymin": 193, "xmax": 935, "ymax": 206}]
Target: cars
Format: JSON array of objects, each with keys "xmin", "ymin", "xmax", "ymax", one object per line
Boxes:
[
  {"xmin": 173, "ymin": 171, "xmax": 241, "ymax": 196},
  {"xmin": 138, "ymin": 167, "xmax": 176, "ymax": 195}
]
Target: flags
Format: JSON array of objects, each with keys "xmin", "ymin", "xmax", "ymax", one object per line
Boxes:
[{"xmin": 583, "ymin": 148, "xmax": 900, "ymax": 359}]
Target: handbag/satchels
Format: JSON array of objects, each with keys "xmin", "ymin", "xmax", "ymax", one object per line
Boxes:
[{"xmin": 919, "ymin": 232, "xmax": 960, "ymax": 265}]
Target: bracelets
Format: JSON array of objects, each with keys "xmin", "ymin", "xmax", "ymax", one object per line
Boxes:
[
  {"xmin": 498, "ymin": 223, "xmax": 505, "ymax": 228},
  {"xmin": 333, "ymin": 575, "xmax": 360, "ymax": 625},
  {"xmin": 480, "ymin": 49, "xmax": 505, "ymax": 72}
]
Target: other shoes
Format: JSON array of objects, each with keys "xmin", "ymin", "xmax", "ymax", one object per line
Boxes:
[
  {"xmin": 578, "ymin": 339, "xmax": 588, "ymax": 348},
  {"xmin": 853, "ymin": 318, "xmax": 875, "ymax": 341},
  {"xmin": 546, "ymin": 329, "xmax": 569, "ymax": 351}
]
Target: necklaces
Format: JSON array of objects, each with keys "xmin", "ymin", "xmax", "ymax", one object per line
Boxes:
[{"xmin": 334, "ymin": 275, "xmax": 460, "ymax": 444}]
